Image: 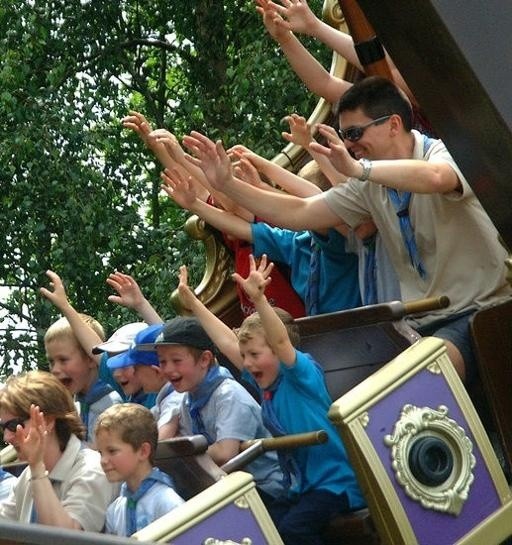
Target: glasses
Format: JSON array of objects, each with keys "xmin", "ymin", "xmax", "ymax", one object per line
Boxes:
[
  {"xmin": 0, "ymin": 417, "xmax": 29, "ymax": 432},
  {"xmin": 339, "ymin": 114, "xmax": 389, "ymax": 141}
]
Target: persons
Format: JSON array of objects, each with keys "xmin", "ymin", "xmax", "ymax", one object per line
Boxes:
[
  {"xmin": 122, "ymin": 1, "xmax": 438, "ymax": 317},
  {"xmin": 0, "ymin": 370, "xmax": 119, "ymax": 535},
  {"xmin": 1, "ymin": 270, "xmax": 186, "ymax": 515},
  {"xmin": 93, "ymin": 402, "xmax": 189, "ymax": 537},
  {"xmin": 181, "ymin": 75, "xmax": 512, "ymax": 386},
  {"xmin": 153, "ymin": 316, "xmax": 289, "ymax": 508},
  {"xmin": 178, "ymin": 252, "xmax": 371, "ymax": 544}
]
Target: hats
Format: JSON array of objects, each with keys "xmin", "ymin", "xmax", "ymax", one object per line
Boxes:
[{"xmin": 91, "ymin": 316, "xmax": 213, "ymax": 369}]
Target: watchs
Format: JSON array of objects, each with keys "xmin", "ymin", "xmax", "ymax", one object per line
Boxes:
[{"xmin": 358, "ymin": 157, "xmax": 373, "ymax": 182}]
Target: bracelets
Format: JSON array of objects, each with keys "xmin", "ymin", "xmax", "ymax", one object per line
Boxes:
[{"xmin": 29, "ymin": 470, "xmax": 49, "ymax": 482}]
